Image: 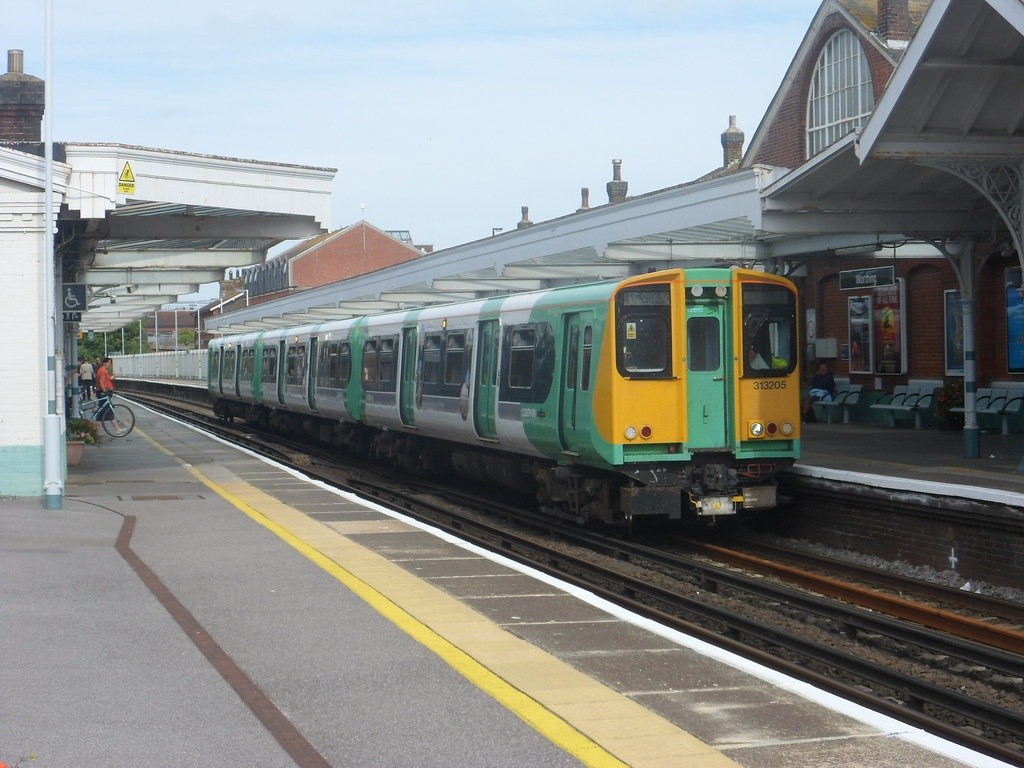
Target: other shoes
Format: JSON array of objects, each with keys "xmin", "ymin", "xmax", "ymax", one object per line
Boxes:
[{"xmin": 115, "ymin": 428, "xmax": 127, "ymax": 434}]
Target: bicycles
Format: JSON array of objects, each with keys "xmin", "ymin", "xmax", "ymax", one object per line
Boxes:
[{"xmin": 69, "ymin": 389, "xmax": 135, "ymax": 438}]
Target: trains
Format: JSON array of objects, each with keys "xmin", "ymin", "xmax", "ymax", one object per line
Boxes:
[{"xmin": 207, "ymin": 264, "xmax": 800, "ymax": 528}]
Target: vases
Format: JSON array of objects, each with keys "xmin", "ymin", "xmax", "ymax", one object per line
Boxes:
[
  {"xmin": 943, "ymin": 415, "xmax": 964, "ymax": 430},
  {"xmin": 66, "ymin": 441, "xmax": 84, "ymax": 466}
]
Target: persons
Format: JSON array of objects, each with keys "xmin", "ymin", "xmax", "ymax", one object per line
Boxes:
[
  {"xmin": 96, "ymin": 358, "xmax": 127, "ymax": 434},
  {"xmin": 64, "ymin": 352, "xmax": 75, "ymax": 418},
  {"xmin": 78, "ymin": 356, "xmax": 101, "ymax": 400},
  {"xmin": 800, "ymin": 364, "xmax": 835, "ymax": 424},
  {"xmin": 749, "ymin": 344, "xmax": 770, "ymax": 369},
  {"xmin": 287, "ymin": 368, "xmax": 294, "ymax": 383}
]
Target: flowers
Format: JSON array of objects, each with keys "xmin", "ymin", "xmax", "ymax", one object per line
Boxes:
[
  {"xmin": 65, "ymin": 418, "xmax": 103, "ymax": 446},
  {"xmin": 932, "ymin": 381, "xmax": 963, "ymax": 418}
]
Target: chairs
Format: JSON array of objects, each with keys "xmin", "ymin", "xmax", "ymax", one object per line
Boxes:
[{"xmin": 811, "ymin": 385, "xmax": 1024, "ymax": 435}]
